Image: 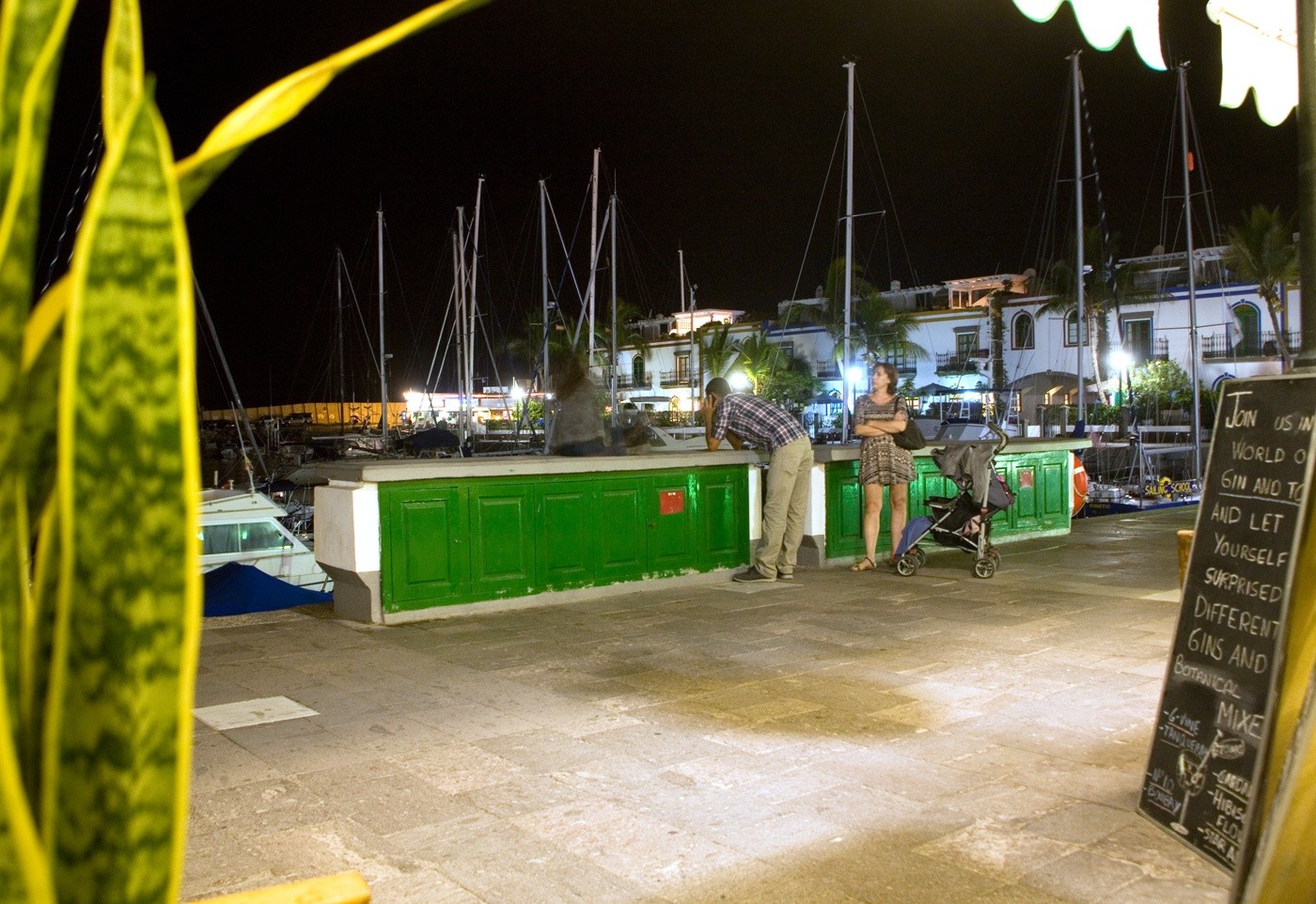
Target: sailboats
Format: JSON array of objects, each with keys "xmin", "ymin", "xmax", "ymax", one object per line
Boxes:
[{"xmin": 196, "ymin": 58, "xmax": 1232, "ymax": 620}]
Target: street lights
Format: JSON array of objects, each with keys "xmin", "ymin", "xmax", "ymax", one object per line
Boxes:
[
  {"xmin": 846, "ymin": 365, "xmax": 862, "ymax": 400},
  {"xmin": 1106, "ymin": 350, "xmax": 1131, "ymax": 408}
]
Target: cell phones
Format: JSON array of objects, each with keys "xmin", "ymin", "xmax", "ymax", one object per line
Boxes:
[{"xmin": 708, "ymin": 393, "xmax": 716, "ymax": 407}]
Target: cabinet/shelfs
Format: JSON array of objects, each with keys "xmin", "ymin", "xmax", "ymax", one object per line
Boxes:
[
  {"xmin": 381, "ymin": 463, "xmax": 751, "ymax": 624},
  {"xmin": 806, "ymin": 451, "xmax": 1075, "ymax": 625}
]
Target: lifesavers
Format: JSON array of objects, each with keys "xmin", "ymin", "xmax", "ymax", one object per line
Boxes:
[{"xmin": 1073, "ymin": 455, "xmax": 1088, "ymax": 516}]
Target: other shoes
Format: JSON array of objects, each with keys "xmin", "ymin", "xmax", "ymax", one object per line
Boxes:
[
  {"xmin": 732, "ymin": 565, "xmax": 776, "ymax": 582},
  {"xmin": 776, "ymin": 566, "xmax": 794, "ymax": 579}
]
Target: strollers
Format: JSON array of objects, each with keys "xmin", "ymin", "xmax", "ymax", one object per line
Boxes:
[{"xmin": 894, "ymin": 421, "xmax": 1016, "ymax": 579}]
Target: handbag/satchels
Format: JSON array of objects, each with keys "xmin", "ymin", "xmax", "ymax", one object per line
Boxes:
[{"xmin": 891, "ymin": 397, "xmax": 927, "ymax": 450}]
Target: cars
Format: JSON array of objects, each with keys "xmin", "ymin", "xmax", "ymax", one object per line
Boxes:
[
  {"xmin": 250, "ymin": 415, "xmax": 277, "ymax": 424},
  {"xmin": 283, "ymin": 412, "xmax": 312, "ymax": 426}
]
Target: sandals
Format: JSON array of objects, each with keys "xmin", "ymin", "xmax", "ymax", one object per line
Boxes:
[
  {"xmin": 851, "ymin": 557, "xmax": 876, "ymax": 571},
  {"xmin": 889, "ymin": 554, "xmax": 899, "ymax": 566}
]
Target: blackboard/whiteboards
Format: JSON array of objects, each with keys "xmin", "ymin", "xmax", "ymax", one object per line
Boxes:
[{"xmin": 1135, "ymin": 372, "xmax": 1316, "ymax": 876}]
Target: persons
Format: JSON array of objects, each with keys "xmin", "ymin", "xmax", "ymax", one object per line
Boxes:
[
  {"xmin": 548, "ymin": 353, "xmax": 608, "ymax": 459},
  {"xmin": 852, "ymin": 362, "xmax": 917, "ymax": 571},
  {"xmin": 701, "ymin": 378, "xmax": 813, "ymax": 582}
]
